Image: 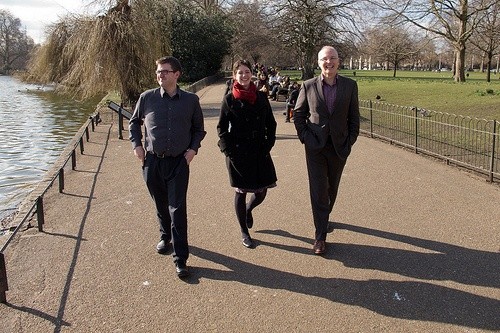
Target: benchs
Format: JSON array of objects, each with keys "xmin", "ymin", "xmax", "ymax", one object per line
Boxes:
[{"xmin": 276, "ymin": 85, "xmax": 290, "ymax": 102}]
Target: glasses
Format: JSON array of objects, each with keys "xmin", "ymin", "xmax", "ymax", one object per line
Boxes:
[
  {"xmin": 319, "ymin": 58, "xmax": 339, "ymax": 62},
  {"xmin": 155, "ymin": 70, "xmax": 176, "ymax": 75}
]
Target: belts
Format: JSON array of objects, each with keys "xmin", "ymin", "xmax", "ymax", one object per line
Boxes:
[{"xmin": 147, "ymin": 151, "xmax": 181, "ymax": 158}]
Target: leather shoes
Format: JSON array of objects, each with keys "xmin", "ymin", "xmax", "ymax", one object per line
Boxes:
[
  {"xmin": 242, "ymin": 233, "xmax": 252, "ymax": 247},
  {"xmin": 246, "ymin": 204, "xmax": 254, "ymax": 228},
  {"xmin": 155, "ymin": 237, "xmax": 171, "ymax": 253},
  {"xmin": 175, "ymin": 261, "xmax": 189, "ymax": 277},
  {"xmin": 313, "ymin": 239, "xmax": 327, "ymax": 254}
]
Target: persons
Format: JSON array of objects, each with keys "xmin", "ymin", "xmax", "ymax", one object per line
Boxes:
[
  {"xmin": 217, "ymin": 60, "xmax": 277, "ymax": 247},
  {"xmin": 225, "ymin": 78, "xmax": 235, "ymax": 95},
  {"xmin": 251, "ymin": 62, "xmax": 299, "ymax": 122},
  {"xmin": 294, "ymin": 46, "xmax": 360, "ymax": 254},
  {"xmin": 129, "ymin": 56, "xmax": 207, "ymax": 276}
]
